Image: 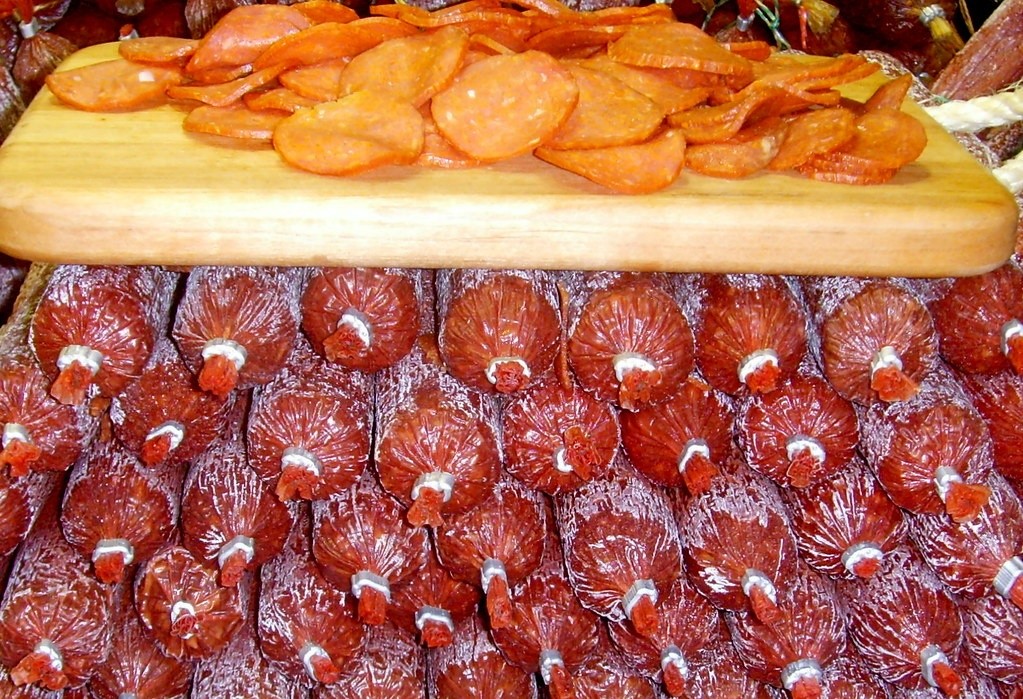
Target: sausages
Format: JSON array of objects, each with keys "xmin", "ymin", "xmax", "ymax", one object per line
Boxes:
[{"xmin": 0, "ymin": 0, "xmax": 1023, "ymax": 699}]
